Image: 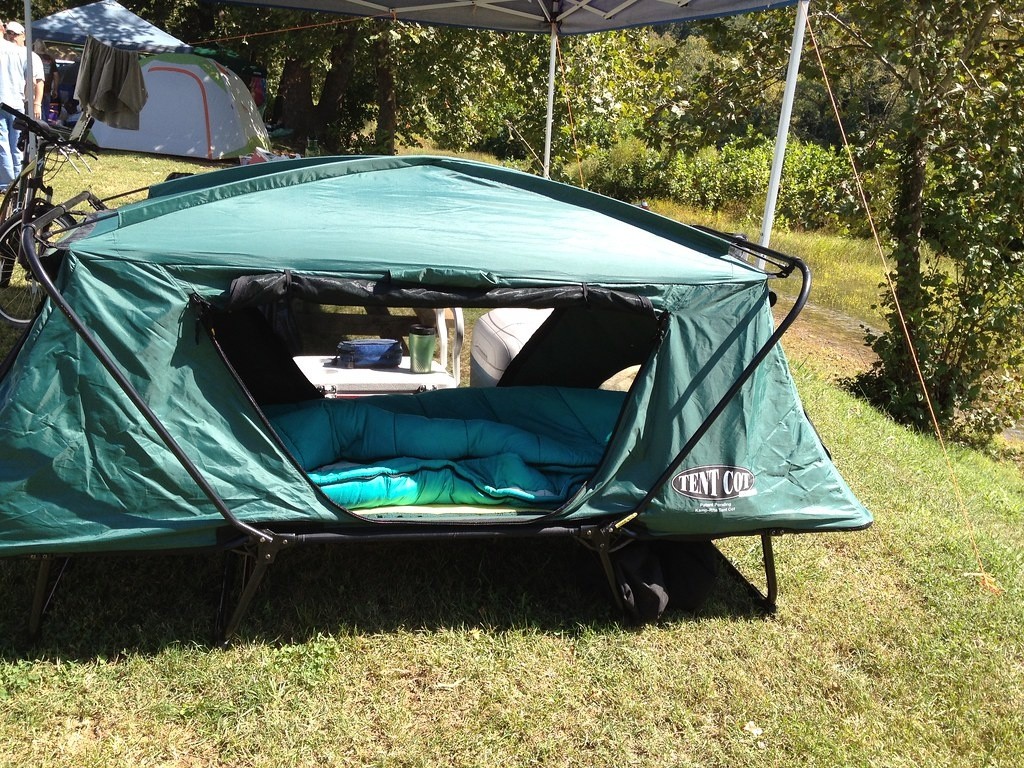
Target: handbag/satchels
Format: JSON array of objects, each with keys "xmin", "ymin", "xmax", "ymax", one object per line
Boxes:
[{"xmin": 332, "ymin": 338, "xmax": 404, "ymax": 368}]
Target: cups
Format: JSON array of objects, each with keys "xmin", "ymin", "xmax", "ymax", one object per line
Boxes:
[{"xmin": 409, "ymin": 324, "xmax": 436, "ymax": 374}]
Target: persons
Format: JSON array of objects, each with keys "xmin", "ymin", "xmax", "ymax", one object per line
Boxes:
[{"xmin": 0, "ymin": 19, "xmax": 59, "ymax": 193}]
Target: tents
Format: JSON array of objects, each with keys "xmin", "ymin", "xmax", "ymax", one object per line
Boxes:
[
  {"xmin": 32, "ymin": 0, "xmax": 194, "ymax": 56},
  {"xmin": 0, "ymin": 158, "xmax": 874, "ymax": 615},
  {"xmin": 68, "ymin": 53, "xmax": 273, "ymax": 160}
]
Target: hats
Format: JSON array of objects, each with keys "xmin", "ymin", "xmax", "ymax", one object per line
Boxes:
[{"xmin": 5, "ymin": 21, "xmax": 24, "ymax": 34}]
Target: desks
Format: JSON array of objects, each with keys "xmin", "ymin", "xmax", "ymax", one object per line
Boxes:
[{"xmin": 294, "ymin": 356, "xmax": 461, "ymax": 399}]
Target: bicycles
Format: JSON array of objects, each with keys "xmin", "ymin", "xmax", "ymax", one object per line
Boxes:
[{"xmin": 0, "ymin": 102, "xmax": 99, "ymax": 330}]
